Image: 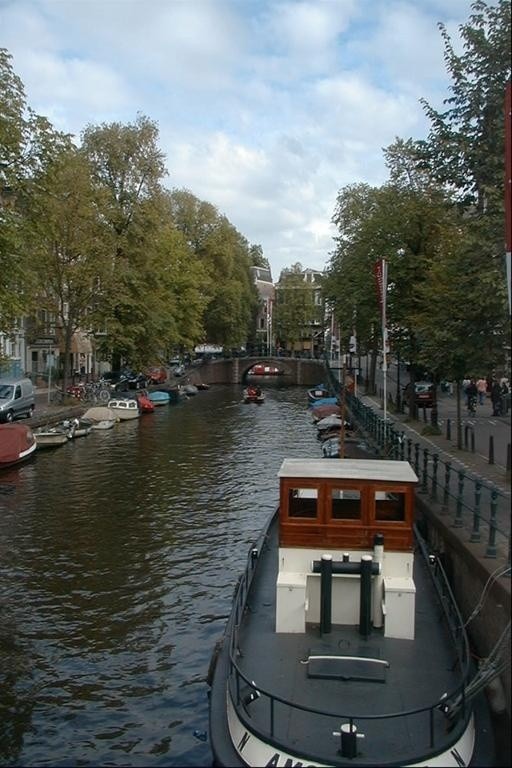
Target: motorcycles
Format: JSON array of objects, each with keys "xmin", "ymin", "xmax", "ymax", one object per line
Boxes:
[{"xmin": 467, "ymin": 397, "xmax": 476, "ymax": 412}]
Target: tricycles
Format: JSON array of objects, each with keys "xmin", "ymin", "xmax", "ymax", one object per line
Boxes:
[{"xmin": 498, "ymin": 394, "xmax": 512, "ymax": 413}]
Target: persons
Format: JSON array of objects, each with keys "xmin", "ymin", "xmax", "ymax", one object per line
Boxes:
[
  {"xmin": 243, "ymin": 384, "xmax": 262, "ymax": 396},
  {"xmin": 80, "ymin": 355, "xmax": 85, "ymax": 373},
  {"xmin": 463, "ymin": 377, "xmax": 508, "ymax": 417}
]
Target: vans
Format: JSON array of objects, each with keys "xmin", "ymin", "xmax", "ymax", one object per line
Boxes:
[{"xmin": 0, "ymin": 376, "xmax": 35, "ymax": 422}]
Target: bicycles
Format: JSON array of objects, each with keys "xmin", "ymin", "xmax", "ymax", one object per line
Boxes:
[{"xmin": 55, "ymin": 374, "xmax": 110, "ymax": 404}]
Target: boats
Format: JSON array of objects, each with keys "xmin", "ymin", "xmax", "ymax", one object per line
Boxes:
[
  {"xmin": 0, "ymin": 384, "xmax": 210, "ymax": 469},
  {"xmin": 240, "ymin": 390, "xmax": 264, "ymax": 403},
  {"xmin": 210, "ymin": 459, "xmax": 497, "ymax": 766},
  {"xmin": 307, "ymin": 388, "xmax": 373, "ymax": 458},
  {"xmin": 248, "ymin": 364, "xmax": 278, "ymax": 375}
]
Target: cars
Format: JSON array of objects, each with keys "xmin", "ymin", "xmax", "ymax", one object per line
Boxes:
[
  {"xmin": 102, "ymin": 360, "xmax": 185, "ymax": 391},
  {"xmin": 403, "ymin": 382, "xmax": 433, "ymax": 407}
]
